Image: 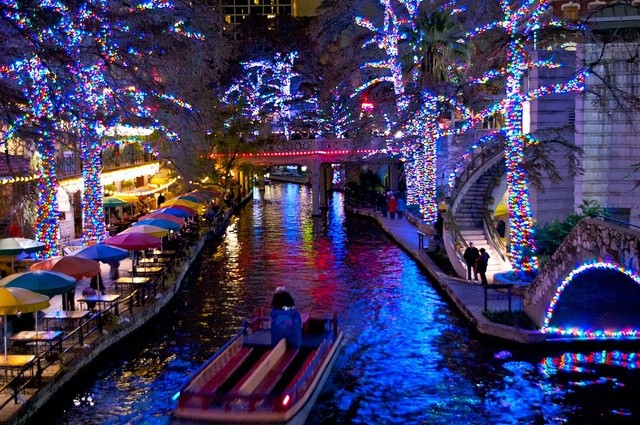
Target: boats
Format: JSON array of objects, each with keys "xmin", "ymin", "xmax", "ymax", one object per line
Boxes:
[{"xmin": 174, "ymin": 306, "xmax": 344, "ymax": 423}]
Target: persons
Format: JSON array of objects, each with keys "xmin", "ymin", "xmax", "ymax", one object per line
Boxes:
[
  {"xmin": 376, "ymin": 190, "xmax": 381, "ymax": 212},
  {"xmin": 388, "ymin": 194, "xmax": 397, "ymax": 220},
  {"xmin": 89, "ymin": 267, "xmax": 106, "ymax": 295},
  {"xmin": 272, "ymin": 285, "xmax": 296, "ymax": 312},
  {"xmin": 81, "ymin": 286, "xmax": 98, "ymax": 310},
  {"xmin": 477, "ymin": 248, "xmax": 490, "ymax": 285},
  {"xmin": 464, "ymin": 242, "xmax": 479, "ymax": 284},
  {"xmin": 110, "ymin": 213, "xmax": 119, "ymax": 224},
  {"xmin": 110, "ymin": 261, "xmax": 120, "ymax": 291},
  {"xmin": 381, "ymin": 192, "xmax": 388, "ymax": 217},
  {"xmin": 344, "ymin": 179, "xmax": 377, "ymax": 212},
  {"xmin": 396, "ymin": 196, "xmax": 406, "ymax": 220}
]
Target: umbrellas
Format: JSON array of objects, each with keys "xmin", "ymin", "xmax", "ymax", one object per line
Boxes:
[
  {"xmin": 160, "ymin": 199, "xmax": 204, "ymax": 224},
  {"xmin": 101, "ymin": 232, "xmax": 162, "ymax": 280},
  {"xmin": 74, "ymin": 242, "xmax": 130, "ymax": 297},
  {"xmin": 103, "ymin": 196, "xmax": 127, "ymax": 226},
  {"xmin": 116, "ymin": 224, "xmax": 169, "ymax": 270},
  {"xmin": 29, "ymin": 255, "xmax": 102, "ymax": 316},
  {"xmin": 152, "ymin": 208, "xmax": 190, "ymax": 218},
  {"xmin": 158, "ymin": 204, "xmax": 198, "ymax": 224},
  {"xmin": 0, "ymin": 269, "xmax": 77, "ymax": 334},
  {"xmin": 177, "ymin": 185, "xmax": 221, "ymax": 203},
  {"xmin": 0, "ymin": 286, "xmax": 50, "ymax": 362},
  {"xmin": 138, "ymin": 213, "xmax": 185, "ymax": 251},
  {"xmin": 113, "ymin": 193, "xmax": 140, "ymax": 218},
  {"xmin": 134, "ymin": 218, "xmax": 181, "ymax": 259},
  {"xmin": 1, "ymin": 236, "xmax": 47, "ymax": 275}
]
